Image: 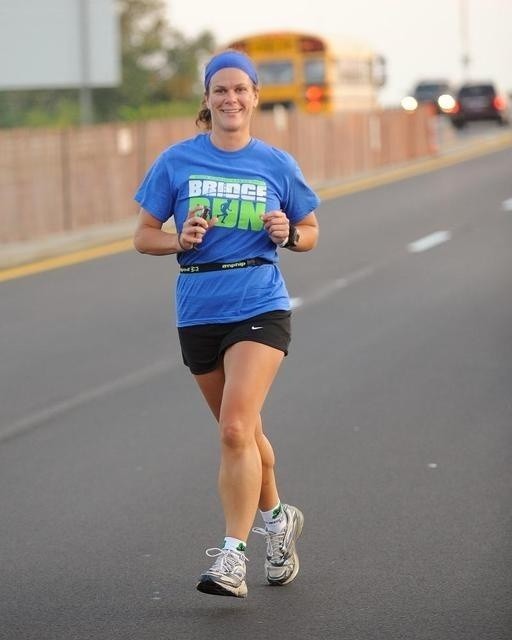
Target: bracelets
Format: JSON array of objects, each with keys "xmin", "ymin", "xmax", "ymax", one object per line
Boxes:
[{"xmin": 177, "ymin": 232, "xmax": 194, "ymax": 252}]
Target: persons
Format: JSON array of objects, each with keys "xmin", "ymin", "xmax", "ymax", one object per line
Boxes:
[{"xmin": 133, "ymin": 49, "xmax": 321, "ymax": 600}]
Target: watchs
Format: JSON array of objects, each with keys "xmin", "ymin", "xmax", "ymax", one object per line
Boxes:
[{"xmin": 279, "ymin": 223, "xmax": 300, "ymax": 250}]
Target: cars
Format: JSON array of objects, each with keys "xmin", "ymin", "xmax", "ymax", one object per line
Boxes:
[
  {"xmin": 401, "ymin": 79, "xmax": 457, "ymax": 115},
  {"xmin": 446, "ymin": 84, "xmax": 508, "ymax": 130}
]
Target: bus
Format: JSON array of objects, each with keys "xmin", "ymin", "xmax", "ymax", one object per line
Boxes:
[{"xmin": 230, "ymin": 30, "xmax": 386, "ymax": 113}]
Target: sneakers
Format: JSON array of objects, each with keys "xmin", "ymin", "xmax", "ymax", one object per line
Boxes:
[
  {"xmin": 252, "ymin": 504, "xmax": 304, "ymax": 586},
  {"xmin": 195, "ymin": 547, "xmax": 249, "ymax": 598}
]
